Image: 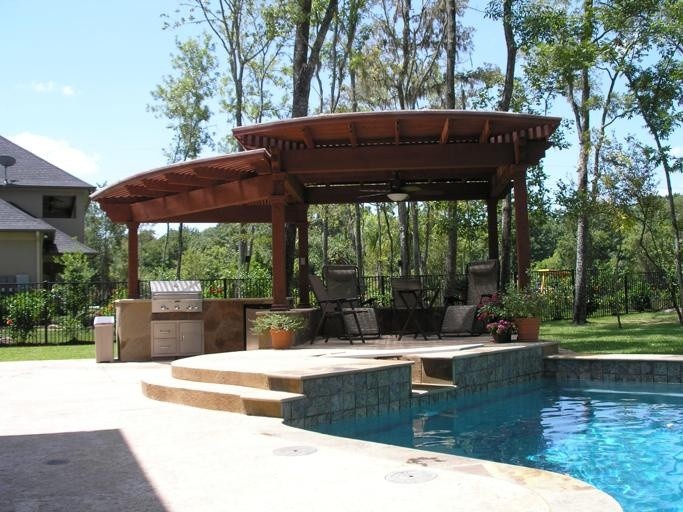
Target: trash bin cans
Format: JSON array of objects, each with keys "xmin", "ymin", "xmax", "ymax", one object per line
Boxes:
[{"xmin": 94, "ymin": 316, "xmax": 114, "ymax": 363}]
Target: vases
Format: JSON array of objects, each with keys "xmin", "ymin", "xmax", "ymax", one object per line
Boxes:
[{"xmin": 491, "ymin": 329, "xmax": 509, "ymax": 343}]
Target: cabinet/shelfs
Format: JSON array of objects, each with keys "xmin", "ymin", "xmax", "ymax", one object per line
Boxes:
[{"xmin": 148, "ymin": 319, "xmax": 206, "ymax": 358}]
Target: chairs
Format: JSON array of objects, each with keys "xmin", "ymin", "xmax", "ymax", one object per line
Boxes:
[
  {"xmin": 439, "ymin": 259, "xmax": 500, "ymax": 339},
  {"xmin": 305, "ymin": 263, "xmax": 384, "ymax": 347},
  {"xmin": 391, "ymin": 275, "xmax": 429, "ymax": 341}
]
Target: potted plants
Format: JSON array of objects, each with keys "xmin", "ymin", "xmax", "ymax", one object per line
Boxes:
[
  {"xmin": 248, "ymin": 313, "xmax": 307, "ymax": 348},
  {"xmin": 500, "ymin": 282, "xmax": 546, "ymax": 341}
]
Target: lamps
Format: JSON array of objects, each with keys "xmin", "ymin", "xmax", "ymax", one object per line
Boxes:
[{"xmin": 388, "ymin": 188, "xmax": 411, "ymax": 202}]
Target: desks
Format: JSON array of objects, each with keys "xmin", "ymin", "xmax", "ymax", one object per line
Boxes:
[{"xmin": 396, "ymin": 285, "xmax": 444, "ymax": 341}]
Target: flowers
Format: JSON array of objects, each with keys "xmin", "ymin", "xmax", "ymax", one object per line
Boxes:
[{"xmin": 475, "ymin": 290, "xmax": 512, "ymax": 331}]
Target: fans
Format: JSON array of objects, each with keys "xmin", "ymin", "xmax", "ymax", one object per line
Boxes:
[{"xmin": 355, "ymin": 170, "xmax": 450, "ymax": 204}]
